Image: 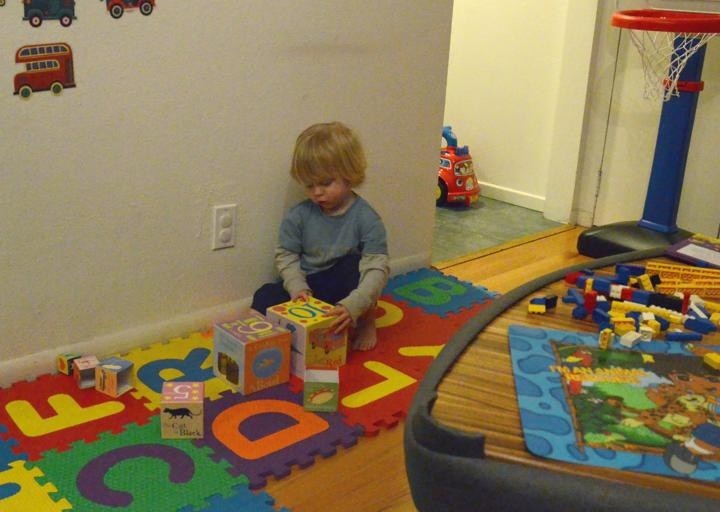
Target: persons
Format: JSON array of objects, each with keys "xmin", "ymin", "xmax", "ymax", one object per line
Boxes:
[{"xmin": 249, "ymin": 120, "xmax": 390, "ymax": 352}]
